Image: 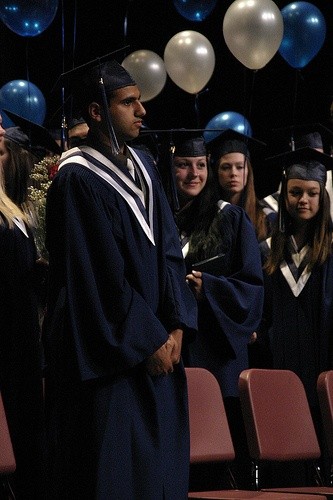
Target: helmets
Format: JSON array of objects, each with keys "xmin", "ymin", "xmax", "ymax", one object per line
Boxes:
[{"xmin": 0, "ymin": 79, "xmax": 50, "ymax": 135}]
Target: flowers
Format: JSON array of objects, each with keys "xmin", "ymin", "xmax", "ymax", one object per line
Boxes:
[{"xmin": 27, "ymin": 153, "xmax": 60, "ymax": 256}]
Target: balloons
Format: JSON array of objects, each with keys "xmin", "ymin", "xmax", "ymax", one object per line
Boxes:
[
  {"xmin": 174, "ymin": 0, "xmax": 216, "ymax": 22},
  {"xmin": 0, "ymin": 0, "xmax": 58, "ymax": 38},
  {"xmin": 278, "ymin": 2, "xmax": 326, "ymax": 70},
  {"xmin": 164, "ymin": 30, "xmax": 215, "ymax": 94},
  {"xmin": 120, "ymin": 50, "xmax": 167, "ymax": 102},
  {"xmin": 0, "ymin": 79, "xmax": 46, "ymax": 125},
  {"xmin": 223, "ymin": 0, "xmax": 284, "ymax": 70},
  {"xmin": 203, "ymin": 112, "xmax": 251, "ymax": 144}
]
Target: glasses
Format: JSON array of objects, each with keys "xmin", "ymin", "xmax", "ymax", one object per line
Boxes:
[{"xmin": 56, "ymin": 136, "xmax": 88, "ymax": 149}]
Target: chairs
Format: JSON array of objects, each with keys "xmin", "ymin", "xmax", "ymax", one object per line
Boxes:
[{"xmin": 185, "ymin": 368, "xmax": 333, "ymax": 500}]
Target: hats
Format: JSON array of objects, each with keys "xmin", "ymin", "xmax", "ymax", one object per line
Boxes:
[
  {"xmin": 278, "ymin": 122, "xmax": 333, "ymax": 150},
  {"xmin": 139, "ymin": 128, "xmax": 225, "ymax": 214},
  {"xmin": 50, "ymin": 109, "xmax": 87, "ymax": 137},
  {"xmin": 4, "ymin": 126, "xmax": 32, "ymax": 147},
  {"xmin": 265, "ymin": 146, "xmax": 333, "ymax": 187},
  {"xmin": 206, "ymin": 129, "xmax": 269, "ymax": 189},
  {"xmin": 60, "ymin": 44, "xmax": 136, "ymax": 155}
]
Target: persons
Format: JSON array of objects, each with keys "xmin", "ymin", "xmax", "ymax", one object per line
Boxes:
[
  {"xmin": 43, "ymin": 95, "xmax": 92, "ymax": 151},
  {"xmin": 140, "ymin": 129, "xmax": 265, "ymax": 400},
  {"xmin": 278, "ymin": 122, "xmax": 333, "ymax": 154},
  {"xmin": 257, "ymin": 146, "xmax": 333, "ymax": 384},
  {"xmin": 40, "ymin": 45, "xmax": 199, "ymax": 500},
  {"xmin": 205, "ymin": 129, "xmax": 276, "ymax": 242},
  {"xmin": 0, "ymin": 126, "xmax": 32, "ymax": 206},
  {"xmin": 0, "ymin": 110, "xmax": 43, "ymax": 383}
]
